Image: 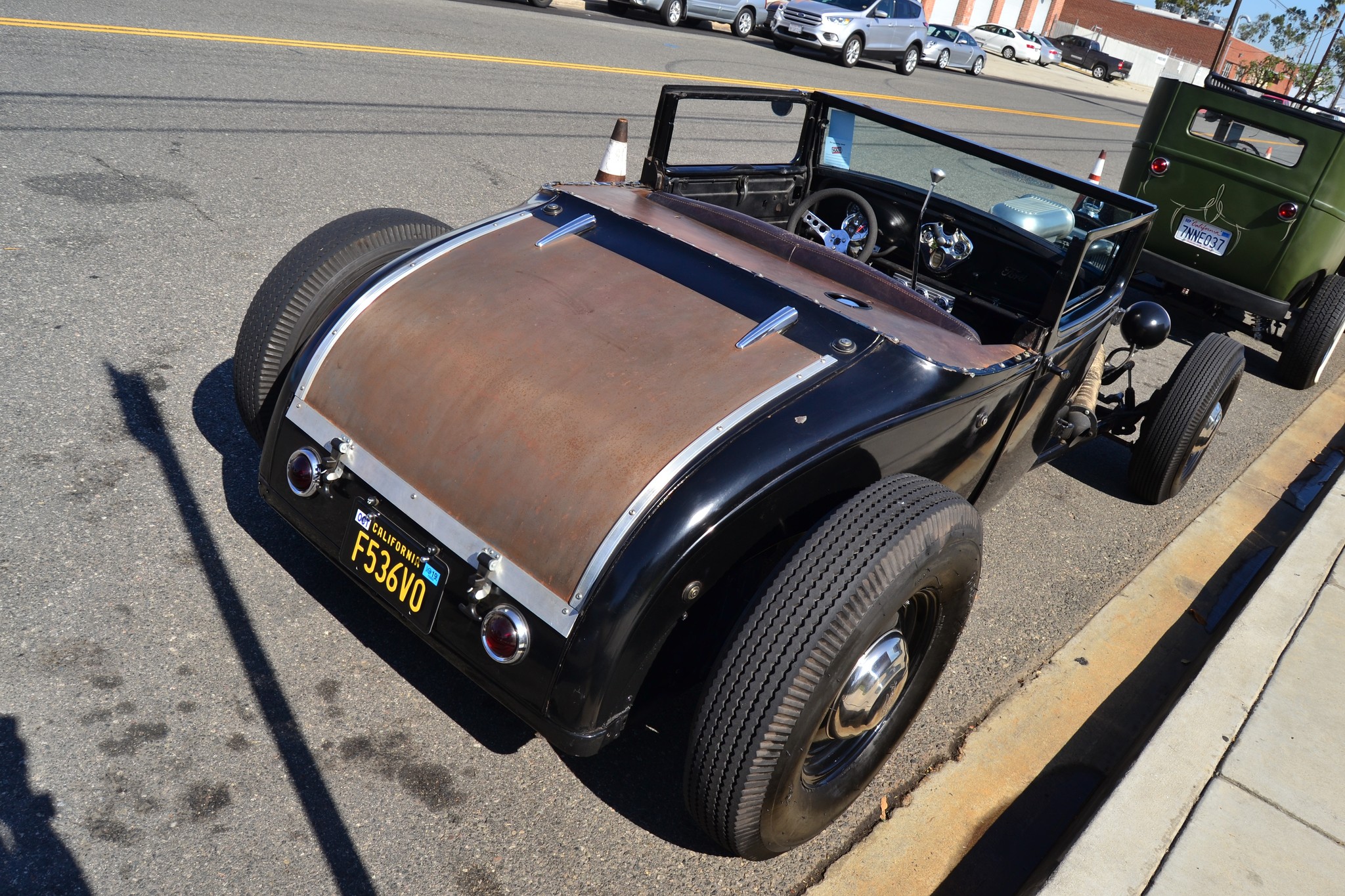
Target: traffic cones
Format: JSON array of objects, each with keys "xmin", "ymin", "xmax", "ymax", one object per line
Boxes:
[
  {"xmin": 1072, "ymin": 150, "xmax": 1107, "ymax": 213},
  {"xmin": 594, "ymin": 118, "xmax": 628, "ymax": 183}
]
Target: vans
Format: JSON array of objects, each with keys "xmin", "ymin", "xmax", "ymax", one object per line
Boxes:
[
  {"xmin": 1260, "ymin": 94, "xmax": 1289, "ymax": 107},
  {"xmin": 1316, "ymin": 111, "xmax": 1338, "ymax": 121}
]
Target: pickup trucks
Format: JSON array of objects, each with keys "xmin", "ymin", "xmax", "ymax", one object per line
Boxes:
[{"xmin": 1043, "ymin": 35, "xmax": 1133, "ymax": 83}]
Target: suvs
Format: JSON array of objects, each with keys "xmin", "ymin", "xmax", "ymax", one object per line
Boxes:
[{"xmin": 763, "ymin": 0, "xmax": 929, "ymax": 77}]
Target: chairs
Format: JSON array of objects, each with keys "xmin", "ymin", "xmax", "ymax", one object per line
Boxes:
[
  {"xmin": 999, "ymin": 29, "xmax": 1003, "ymax": 33},
  {"xmin": 1076, "ymin": 41, "xmax": 1082, "ymax": 46}
]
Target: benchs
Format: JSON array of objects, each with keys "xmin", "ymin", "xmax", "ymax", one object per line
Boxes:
[{"xmin": 645, "ymin": 191, "xmax": 981, "ymax": 346}]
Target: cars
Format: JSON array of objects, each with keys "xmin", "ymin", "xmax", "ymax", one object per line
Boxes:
[
  {"xmin": 1116, "ymin": 73, "xmax": 1345, "ymax": 391},
  {"xmin": 1021, "ymin": 31, "xmax": 1063, "ymax": 67},
  {"xmin": 949, "ymin": 22, "xmax": 1042, "ymax": 63},
  {"xmin": 920, "ymin": 23, "xmax": 987, "ymax": 76},
  {"xmin": 232, "ymin": 90, "xmax": 1248, "ymax": 862},
  {"xmin": 583, "ymin": 0, "xmax": 768, "ymax": 38}
]
